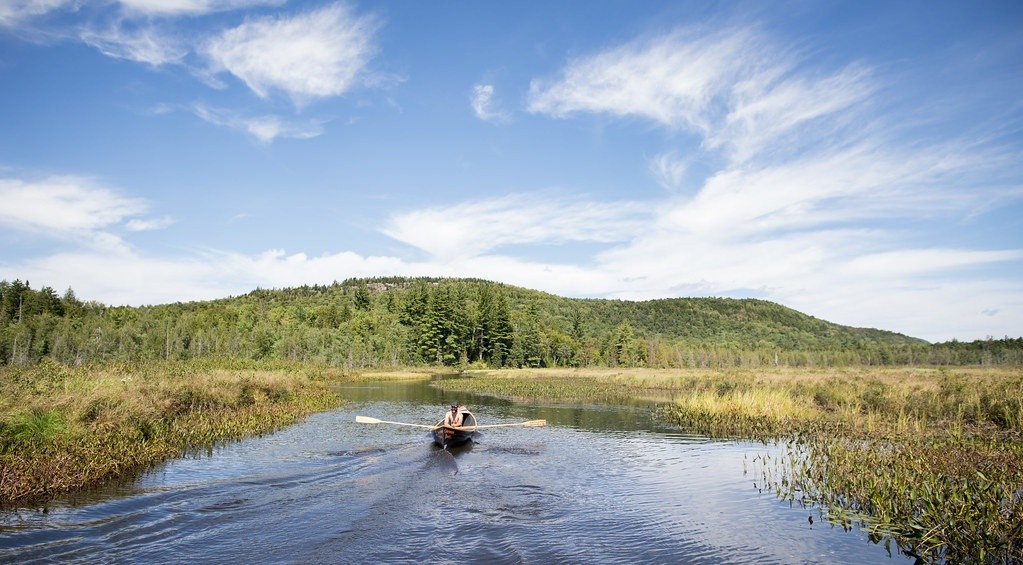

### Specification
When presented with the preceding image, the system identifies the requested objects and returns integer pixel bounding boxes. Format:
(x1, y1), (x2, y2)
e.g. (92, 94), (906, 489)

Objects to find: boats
(430, 404), (479, 449)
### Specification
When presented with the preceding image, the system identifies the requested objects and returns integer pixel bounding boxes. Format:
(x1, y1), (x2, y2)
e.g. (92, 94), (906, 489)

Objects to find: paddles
(354, 415), (436, 428)
(455, 420), (547, 429)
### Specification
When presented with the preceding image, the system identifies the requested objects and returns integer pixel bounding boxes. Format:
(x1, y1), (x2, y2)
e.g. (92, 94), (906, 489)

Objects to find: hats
(451, 402), (459, 408)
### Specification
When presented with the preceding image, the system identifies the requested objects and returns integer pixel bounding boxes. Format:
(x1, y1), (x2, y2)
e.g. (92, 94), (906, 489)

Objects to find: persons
(444, 401), (464, 427)
